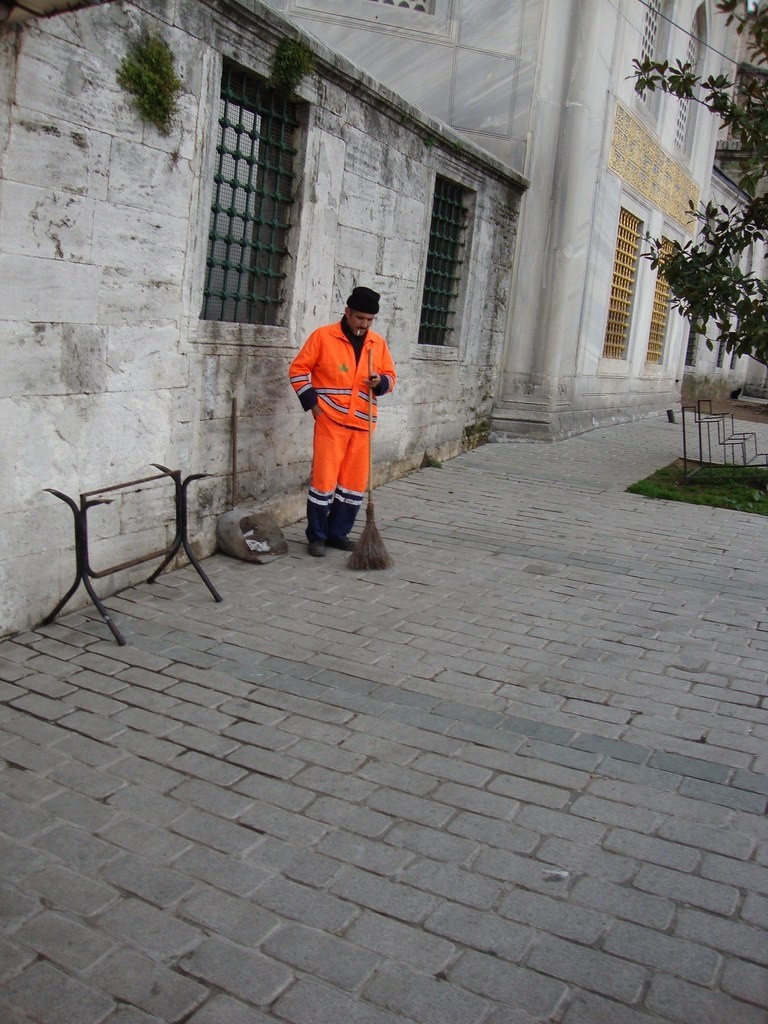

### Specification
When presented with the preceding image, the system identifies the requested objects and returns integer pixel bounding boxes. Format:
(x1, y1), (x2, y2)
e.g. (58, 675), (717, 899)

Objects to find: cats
(731, 388), (742, 399)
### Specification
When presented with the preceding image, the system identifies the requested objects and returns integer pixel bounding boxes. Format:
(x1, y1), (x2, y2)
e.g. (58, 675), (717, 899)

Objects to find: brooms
(345, 348), (395, 572)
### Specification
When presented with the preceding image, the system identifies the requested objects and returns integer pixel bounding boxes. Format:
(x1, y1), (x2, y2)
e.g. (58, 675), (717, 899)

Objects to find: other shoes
(326, 535), (358, 551)
(307, 539), (327, 556)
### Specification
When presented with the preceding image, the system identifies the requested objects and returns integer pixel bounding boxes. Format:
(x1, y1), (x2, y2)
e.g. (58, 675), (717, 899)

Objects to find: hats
(347, 286), (380, 314)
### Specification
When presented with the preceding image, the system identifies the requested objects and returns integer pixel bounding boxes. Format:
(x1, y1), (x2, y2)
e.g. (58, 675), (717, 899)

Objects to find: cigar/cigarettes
(356, 328), (360, 335)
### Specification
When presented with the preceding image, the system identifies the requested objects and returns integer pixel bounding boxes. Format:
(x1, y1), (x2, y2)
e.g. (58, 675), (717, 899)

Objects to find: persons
(289, 285), (397, 556)
